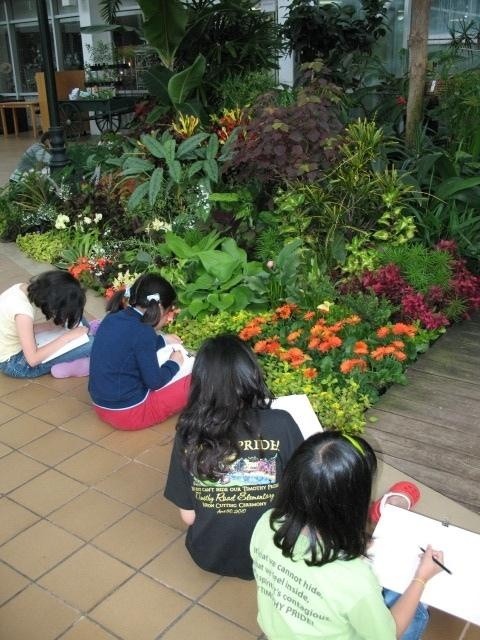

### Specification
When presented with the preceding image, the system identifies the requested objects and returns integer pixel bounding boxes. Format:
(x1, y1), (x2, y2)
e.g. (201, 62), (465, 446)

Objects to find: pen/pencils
(418, 545), (453, 576)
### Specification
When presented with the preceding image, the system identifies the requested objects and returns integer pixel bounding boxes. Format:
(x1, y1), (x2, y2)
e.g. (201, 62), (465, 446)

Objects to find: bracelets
(60, 337), (68, 343)
(412, 578), (425, 585)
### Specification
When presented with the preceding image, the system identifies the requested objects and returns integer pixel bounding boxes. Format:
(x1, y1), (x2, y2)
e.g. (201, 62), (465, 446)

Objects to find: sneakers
(50, 357), (91, 379)
(89, 319), (101, 336)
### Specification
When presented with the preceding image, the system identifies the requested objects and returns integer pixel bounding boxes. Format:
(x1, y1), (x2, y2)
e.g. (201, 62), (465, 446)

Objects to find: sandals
(369, 480), (420, 524)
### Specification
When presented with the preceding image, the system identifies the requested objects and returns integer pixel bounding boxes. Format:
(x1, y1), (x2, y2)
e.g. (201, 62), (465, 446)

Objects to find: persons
(88, 273), (193, 431)
(9, 132), (52, 183)
(0, 270), (101, 379)
(249, 430), (444, 640)
(162, 334), (304, 581)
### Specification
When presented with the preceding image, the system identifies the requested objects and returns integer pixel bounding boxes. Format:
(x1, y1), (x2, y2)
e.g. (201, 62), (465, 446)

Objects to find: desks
(0, 101), (42, 139)
